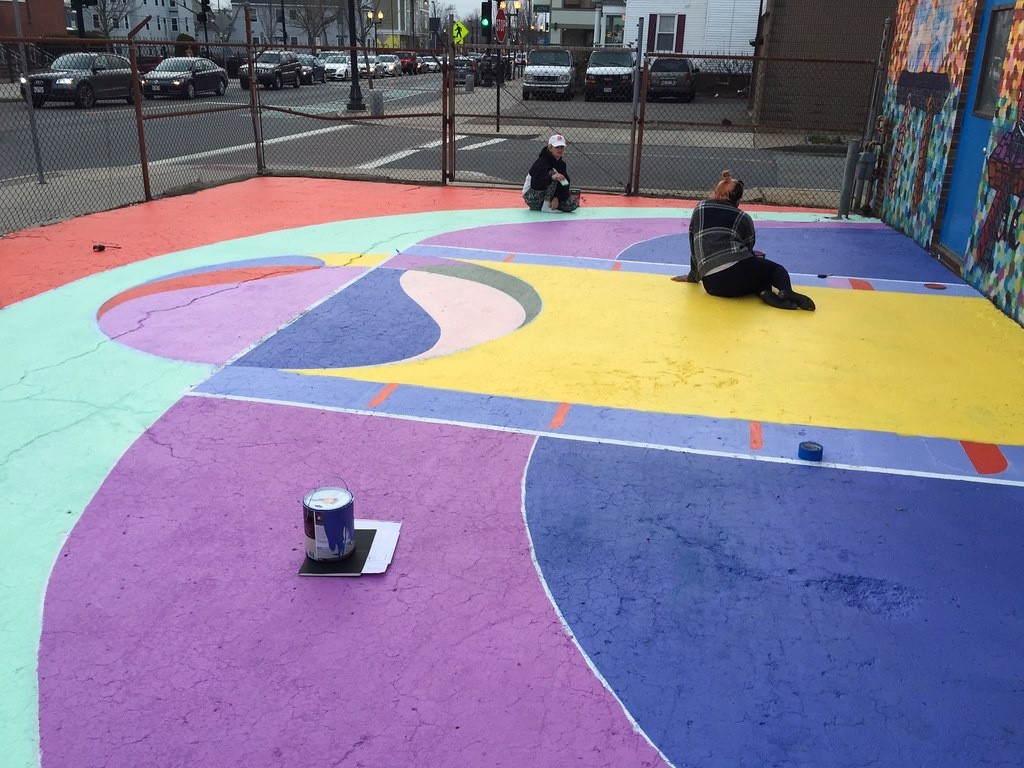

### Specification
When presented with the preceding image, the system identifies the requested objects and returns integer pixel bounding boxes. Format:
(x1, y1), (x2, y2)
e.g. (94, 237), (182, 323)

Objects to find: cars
(139, 57), (228, 100)
(358, 56), (386, 78)
(20, 53), (143, 109)
(326, 58), (352, 80)
(296, 52), (327, 84)
(438, 57), (449, 69)
(378, 55), (402, 77)
(317, 51), (345, 65)
(416, 57), (427, 75)
(425, 57), (441, 72)
(454, 51), (526, 86)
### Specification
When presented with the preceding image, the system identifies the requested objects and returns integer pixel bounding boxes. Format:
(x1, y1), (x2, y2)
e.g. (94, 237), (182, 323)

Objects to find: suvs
(445, 58), (478, 88)
(238, 49), (303, 91)
(397, 52), (418, 75)
(648, 57), (701, 102)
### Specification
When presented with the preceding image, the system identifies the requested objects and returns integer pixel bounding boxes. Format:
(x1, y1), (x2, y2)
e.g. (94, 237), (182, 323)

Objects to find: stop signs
(495, 8), (506, 43)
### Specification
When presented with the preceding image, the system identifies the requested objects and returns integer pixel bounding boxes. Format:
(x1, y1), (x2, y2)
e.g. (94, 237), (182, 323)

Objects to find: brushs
(553, 167), (569, 186)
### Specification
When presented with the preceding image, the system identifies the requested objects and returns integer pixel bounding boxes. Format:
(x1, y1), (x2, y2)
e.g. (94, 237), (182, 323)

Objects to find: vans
(522, 49), (579, 101)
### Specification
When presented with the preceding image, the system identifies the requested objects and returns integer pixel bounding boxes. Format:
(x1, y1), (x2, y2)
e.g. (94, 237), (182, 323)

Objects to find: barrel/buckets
(302, 476), (356, 560)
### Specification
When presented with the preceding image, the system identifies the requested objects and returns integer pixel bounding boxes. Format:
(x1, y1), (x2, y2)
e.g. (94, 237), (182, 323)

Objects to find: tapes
(798, 440), (824, 461)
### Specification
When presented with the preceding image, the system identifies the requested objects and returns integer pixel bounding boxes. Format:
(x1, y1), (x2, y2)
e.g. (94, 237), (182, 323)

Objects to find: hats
(548, 135), (566, 148)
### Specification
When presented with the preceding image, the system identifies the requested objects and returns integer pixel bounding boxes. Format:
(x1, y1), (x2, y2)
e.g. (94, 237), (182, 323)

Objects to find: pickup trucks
(584, 50), (637, 103)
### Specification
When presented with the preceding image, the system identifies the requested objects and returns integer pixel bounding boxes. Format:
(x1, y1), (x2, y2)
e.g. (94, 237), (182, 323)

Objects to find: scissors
(818, 273), (833, 278)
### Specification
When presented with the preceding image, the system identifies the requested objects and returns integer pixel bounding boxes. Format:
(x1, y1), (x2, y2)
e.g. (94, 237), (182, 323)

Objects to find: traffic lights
(85, 0), (98, 8)
(201, 0), (211, 13)
(481, 2), (491, 37)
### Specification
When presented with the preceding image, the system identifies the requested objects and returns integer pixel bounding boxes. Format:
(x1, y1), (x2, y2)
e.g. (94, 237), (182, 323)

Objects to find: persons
(670, 170), (815, 311)
(521, 135), (570, 213)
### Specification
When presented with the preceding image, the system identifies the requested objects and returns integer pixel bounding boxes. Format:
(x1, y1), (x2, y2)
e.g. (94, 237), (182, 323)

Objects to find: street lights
(368, 10), (384, 57)
(499, 0), (521, 81)
